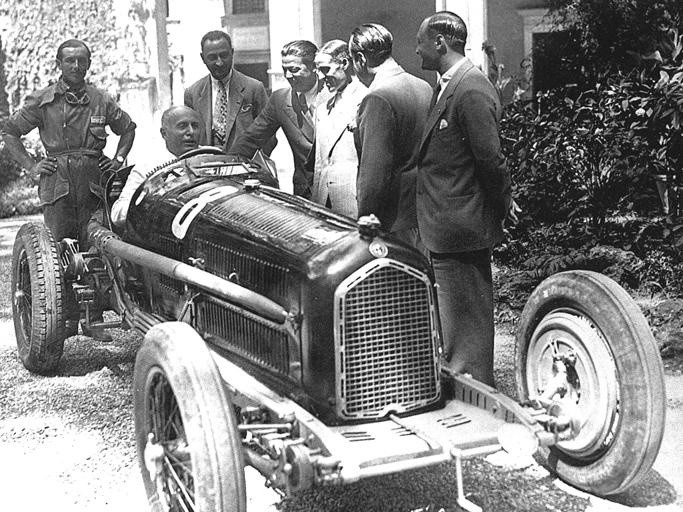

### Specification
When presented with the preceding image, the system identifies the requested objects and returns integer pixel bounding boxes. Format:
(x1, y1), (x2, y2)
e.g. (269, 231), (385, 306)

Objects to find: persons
(348, 24), (433, 261)
(3, 39), (137, 252)
(415, 12), (522, 389)
(110, 106), (202, 230)
(227, 24), (368, 221)
(183, 31), (278, 159)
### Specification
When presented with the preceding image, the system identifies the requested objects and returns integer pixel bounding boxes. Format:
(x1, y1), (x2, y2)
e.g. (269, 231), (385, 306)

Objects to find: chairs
(104, 164), (136, 236)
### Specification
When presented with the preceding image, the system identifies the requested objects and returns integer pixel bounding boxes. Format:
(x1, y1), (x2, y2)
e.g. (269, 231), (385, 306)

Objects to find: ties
(299, 94), (308, 114)
(213, 81), (228, 144)
(428, 78), (441, 116)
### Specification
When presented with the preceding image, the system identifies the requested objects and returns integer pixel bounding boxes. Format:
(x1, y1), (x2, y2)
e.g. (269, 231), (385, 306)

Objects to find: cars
(11, 145), (667, 512)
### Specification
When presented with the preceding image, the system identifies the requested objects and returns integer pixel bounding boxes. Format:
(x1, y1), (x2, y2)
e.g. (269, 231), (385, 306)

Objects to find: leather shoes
(64, 318), (112, 342)
